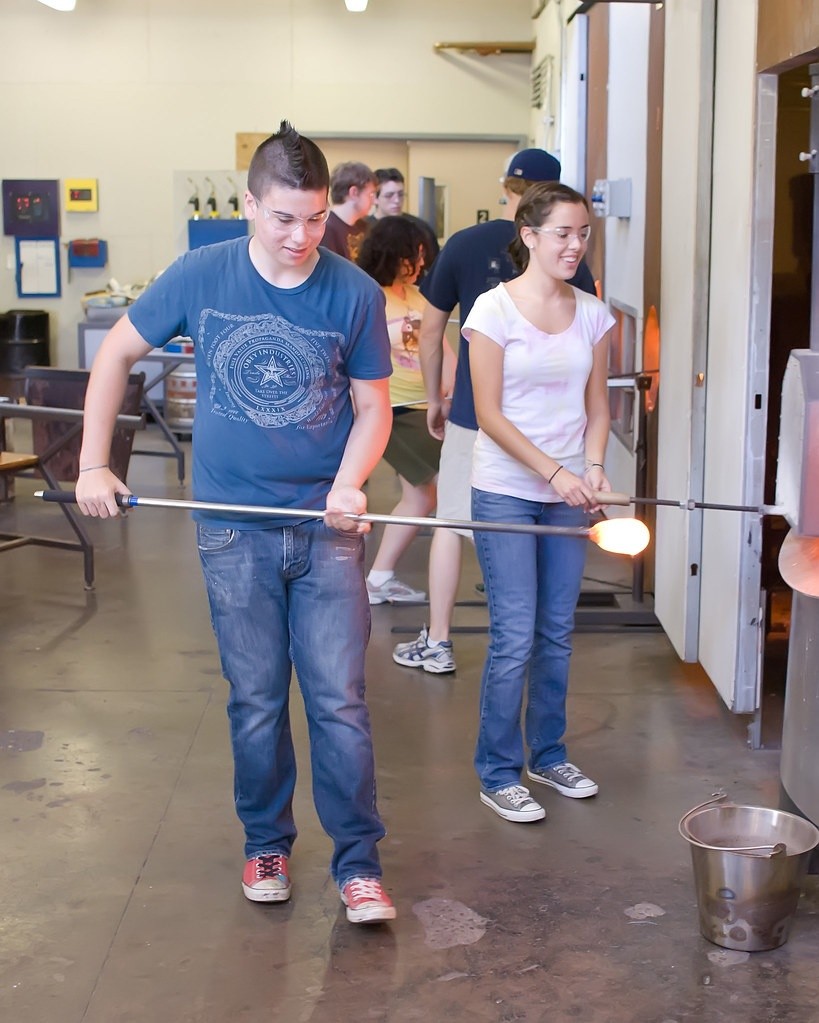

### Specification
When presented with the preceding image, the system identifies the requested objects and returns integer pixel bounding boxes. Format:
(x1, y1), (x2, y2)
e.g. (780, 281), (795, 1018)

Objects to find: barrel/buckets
(678, 787), (819, 951)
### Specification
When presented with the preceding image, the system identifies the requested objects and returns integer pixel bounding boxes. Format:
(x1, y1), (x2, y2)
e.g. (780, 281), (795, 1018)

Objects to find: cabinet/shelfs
(77, 319), (165, 417)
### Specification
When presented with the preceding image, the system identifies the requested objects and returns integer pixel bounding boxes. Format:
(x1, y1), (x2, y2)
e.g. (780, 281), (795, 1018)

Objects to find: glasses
(530, 226), (592, 244)
(254, 198), (331, 231)
(357, 190), (376, 200)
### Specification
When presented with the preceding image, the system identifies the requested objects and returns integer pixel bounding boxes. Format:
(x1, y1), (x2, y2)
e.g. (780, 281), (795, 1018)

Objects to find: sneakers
(527, 763), (599, 799)
(393, 623), (457, 674)
(242, 854), (291, 903)
(366, 576), (427, 605)
(339, 876), (396, 925)
(478, 785), (546, 822)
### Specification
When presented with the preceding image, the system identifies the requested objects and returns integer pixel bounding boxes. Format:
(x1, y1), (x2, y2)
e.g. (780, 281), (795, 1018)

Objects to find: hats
(506, 148), (562, 182)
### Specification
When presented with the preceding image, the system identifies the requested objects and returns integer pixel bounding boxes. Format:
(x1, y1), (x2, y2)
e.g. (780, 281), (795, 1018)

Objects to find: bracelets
(79, 464), (107, 472)
(593, 464), (604, 469)
(548, 466), (563, 483)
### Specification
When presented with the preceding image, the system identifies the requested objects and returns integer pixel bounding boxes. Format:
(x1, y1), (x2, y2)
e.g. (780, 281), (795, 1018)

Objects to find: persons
(76, 120), (397, 924)
(353, 215), (458, 605)
(460, 182), (616, 822)
(320, 160), (406, 261)
(391, 148), (598, 674)
